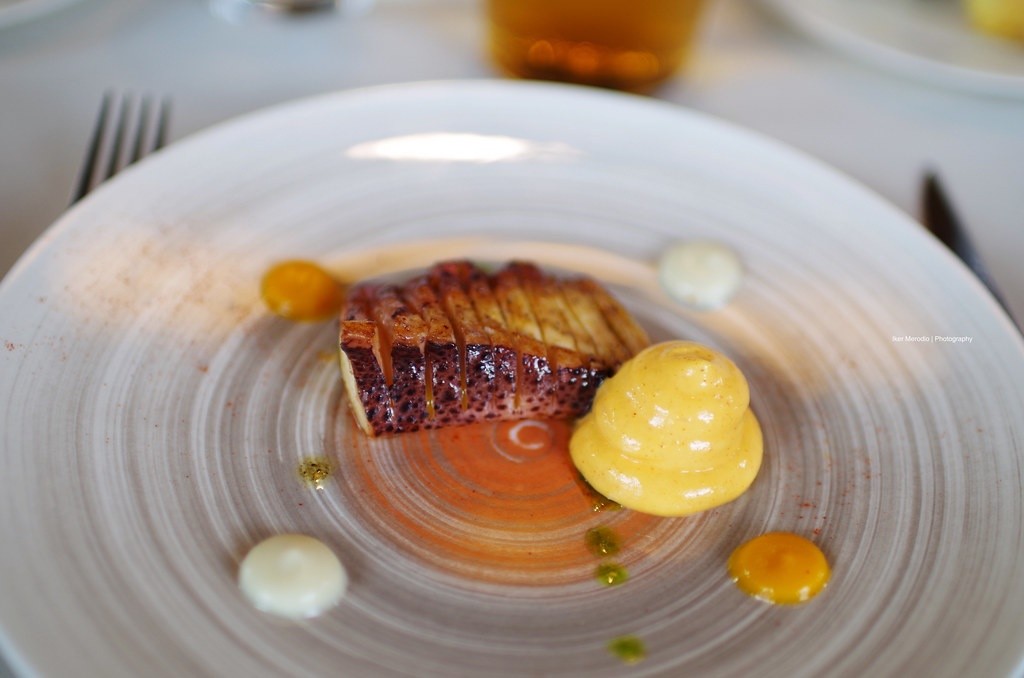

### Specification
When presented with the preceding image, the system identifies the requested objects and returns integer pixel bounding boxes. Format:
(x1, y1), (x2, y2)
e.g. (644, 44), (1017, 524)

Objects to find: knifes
(920, 168), (1023, 341)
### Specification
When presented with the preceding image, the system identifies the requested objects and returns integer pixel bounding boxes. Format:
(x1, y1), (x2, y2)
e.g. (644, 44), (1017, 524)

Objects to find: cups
(473, 0), (711, 97)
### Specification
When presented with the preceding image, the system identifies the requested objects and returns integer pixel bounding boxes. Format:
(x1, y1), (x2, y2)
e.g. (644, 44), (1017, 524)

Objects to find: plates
(760, 0), (1024, 103)
(0, 81), (1023, 678)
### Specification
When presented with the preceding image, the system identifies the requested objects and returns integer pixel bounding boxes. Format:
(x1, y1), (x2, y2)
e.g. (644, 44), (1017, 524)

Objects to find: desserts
(568, 338), (765, 520)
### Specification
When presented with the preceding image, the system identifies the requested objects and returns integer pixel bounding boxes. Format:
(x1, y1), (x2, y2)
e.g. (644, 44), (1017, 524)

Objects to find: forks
(57, 89), (176, 212)
